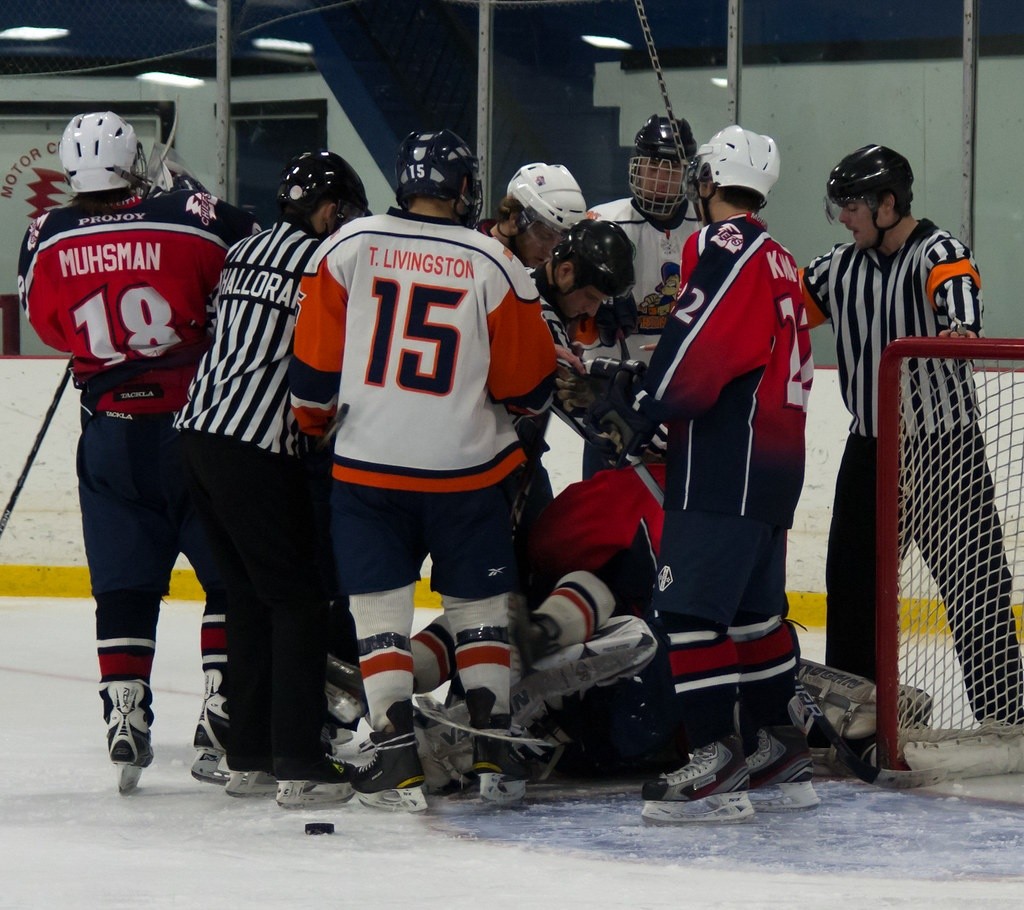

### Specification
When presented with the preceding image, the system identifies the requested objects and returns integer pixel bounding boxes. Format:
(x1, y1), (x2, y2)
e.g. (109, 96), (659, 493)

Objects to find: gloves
(554, 360), (591, 416)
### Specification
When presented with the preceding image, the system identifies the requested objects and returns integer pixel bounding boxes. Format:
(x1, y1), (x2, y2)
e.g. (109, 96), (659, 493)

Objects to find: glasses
(526, 219), (565, 246)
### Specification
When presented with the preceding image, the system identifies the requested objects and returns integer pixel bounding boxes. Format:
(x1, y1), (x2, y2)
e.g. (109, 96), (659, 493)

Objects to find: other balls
(305, 823), (334, 835)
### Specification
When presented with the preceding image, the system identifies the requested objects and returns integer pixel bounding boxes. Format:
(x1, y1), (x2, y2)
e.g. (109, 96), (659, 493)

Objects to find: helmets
(633, 114), (697, 163)
(274, 147), (370, 226)
(693, 124), (782, 201)
(558, 218), (638, 301)
(57, 110), (140, 193)
(826, 144), (913, 211)
(393, 127), (481, 200)
(506, 161), (587, 232)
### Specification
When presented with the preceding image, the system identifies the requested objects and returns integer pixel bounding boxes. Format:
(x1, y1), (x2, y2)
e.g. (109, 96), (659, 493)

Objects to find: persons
(15, 109), (260, 794)
(476, 159), (588, 267)
(402, 460), (690, 695)
(165, 148), (381, 809)
(509, 217), (637, 566)
(629, 126), (822, 821)
(793, 143), (1024, 729)
(289, 127), (563, 814)
(585, 115), (720, 366)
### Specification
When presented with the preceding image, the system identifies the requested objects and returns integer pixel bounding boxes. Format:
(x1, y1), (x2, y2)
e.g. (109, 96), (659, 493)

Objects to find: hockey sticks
(0, 92), (180, 537)
(617, 328), (631, 364)
(632, 0), (702, 221)
(552, 402), (623, 453)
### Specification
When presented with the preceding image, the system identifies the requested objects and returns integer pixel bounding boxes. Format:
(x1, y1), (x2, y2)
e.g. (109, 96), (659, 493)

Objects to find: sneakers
(189, 668), (229, 786)
(348, 697), (428, 814)
(219, 750), (279, 800)
(464, 686), (534, 804)
(273, 751), (358, 811)
(741, 723), (819, 813)
(641, 731), (754, 826)
(99, 678), (154, 796)
